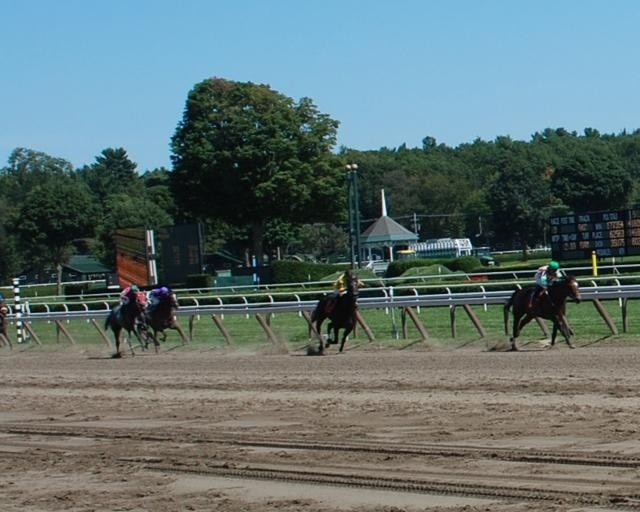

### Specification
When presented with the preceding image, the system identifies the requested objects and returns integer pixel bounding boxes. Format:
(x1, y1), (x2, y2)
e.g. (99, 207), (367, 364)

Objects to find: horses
(105, 290), (189, 359)
(0, 304), (13, 352)
(310, 269), (360, 353)
(503, 276), (582, 351)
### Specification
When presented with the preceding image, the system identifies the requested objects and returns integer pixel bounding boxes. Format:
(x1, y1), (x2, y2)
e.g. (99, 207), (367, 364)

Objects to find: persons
(0, 292), (10, 315)
(534, 260), (559, 302)
(147, 285), (170, 309)
(332, 270), (364, 308)
(120, 284), (150, 347)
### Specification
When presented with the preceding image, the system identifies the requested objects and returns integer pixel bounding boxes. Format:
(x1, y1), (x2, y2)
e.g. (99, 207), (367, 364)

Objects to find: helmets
(161, 286), (169, 294)
(131, 284), (138, 292)
(549, 260), (560, 269)
